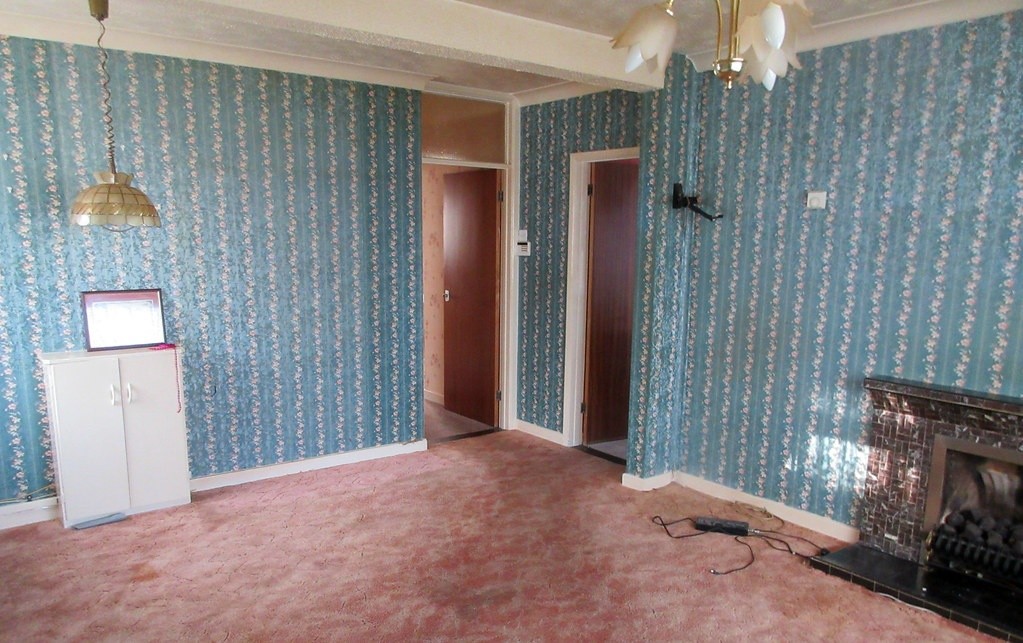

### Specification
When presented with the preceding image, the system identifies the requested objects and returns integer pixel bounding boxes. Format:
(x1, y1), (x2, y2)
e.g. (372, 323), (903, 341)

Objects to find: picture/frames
(82, 288), (168, 352)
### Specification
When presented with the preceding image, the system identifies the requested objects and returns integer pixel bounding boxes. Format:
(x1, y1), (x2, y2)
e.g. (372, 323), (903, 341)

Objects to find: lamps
(607, 0), (814, 91)
(68, 0), (161, 232)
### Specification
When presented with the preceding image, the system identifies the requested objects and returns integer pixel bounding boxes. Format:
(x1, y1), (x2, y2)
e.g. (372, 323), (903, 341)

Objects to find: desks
(37, 343), (192, 529)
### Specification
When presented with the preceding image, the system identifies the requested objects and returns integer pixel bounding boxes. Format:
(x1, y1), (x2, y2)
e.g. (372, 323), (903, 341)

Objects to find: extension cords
(694, 516), (749, 536)
